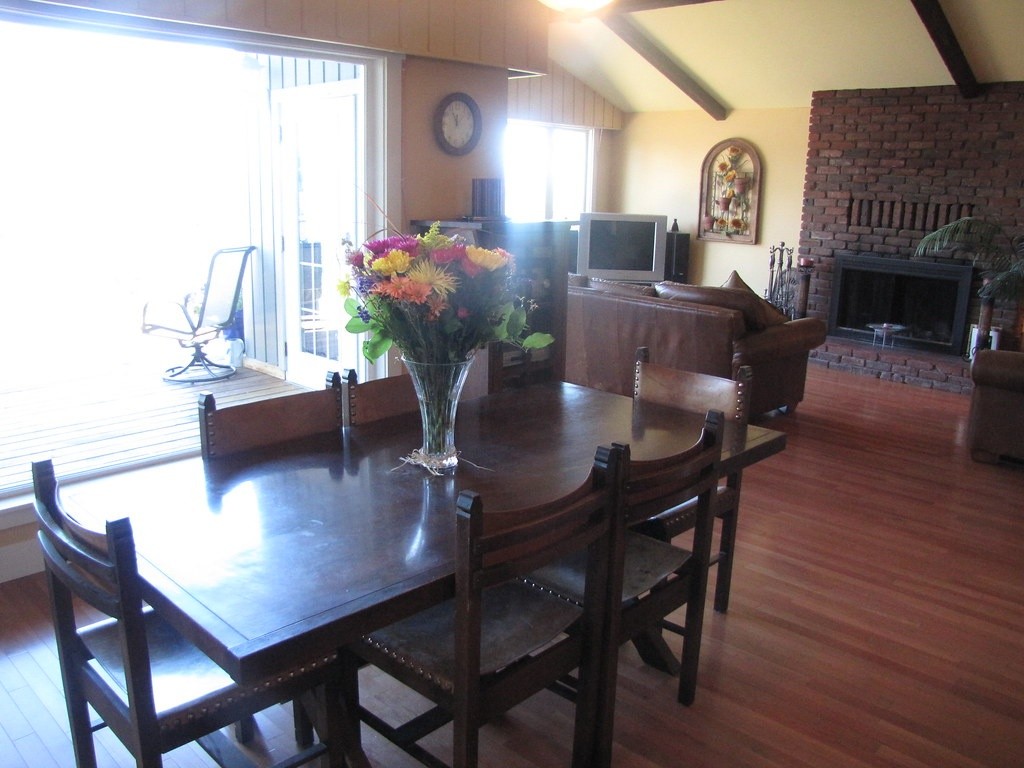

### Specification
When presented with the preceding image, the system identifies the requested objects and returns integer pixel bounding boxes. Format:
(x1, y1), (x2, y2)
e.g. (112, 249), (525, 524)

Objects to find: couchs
(963, 350), (1024, 464)
(563, 265), (828, 421)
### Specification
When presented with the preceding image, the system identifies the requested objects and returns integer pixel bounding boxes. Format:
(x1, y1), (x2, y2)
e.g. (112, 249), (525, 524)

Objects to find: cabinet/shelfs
(410, 219), (579, 396)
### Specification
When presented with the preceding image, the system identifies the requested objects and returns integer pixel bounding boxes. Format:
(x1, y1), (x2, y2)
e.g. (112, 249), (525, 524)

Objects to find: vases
(399, 351), (475, 473)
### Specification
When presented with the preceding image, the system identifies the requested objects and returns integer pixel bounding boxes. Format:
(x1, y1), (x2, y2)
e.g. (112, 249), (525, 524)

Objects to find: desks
(59, 377), (792, 768)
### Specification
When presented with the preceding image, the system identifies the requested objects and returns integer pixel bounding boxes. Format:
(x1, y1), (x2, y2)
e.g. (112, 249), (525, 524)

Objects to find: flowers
(342, 223), (548, 371)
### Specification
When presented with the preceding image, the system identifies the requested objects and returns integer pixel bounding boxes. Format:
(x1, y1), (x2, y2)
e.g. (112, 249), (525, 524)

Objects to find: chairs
(138, 243), (258, 385)
(342, 367), (430, 427)
(520, 406), (735, 768)
(345, 432), (630, 767)
(198, 370), (342, 453)
(626, 347), (757, 611)
(32, 450), (361, 768)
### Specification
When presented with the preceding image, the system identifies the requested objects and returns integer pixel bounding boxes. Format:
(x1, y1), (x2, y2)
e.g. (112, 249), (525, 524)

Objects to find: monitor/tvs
(577, 212), (668, 285)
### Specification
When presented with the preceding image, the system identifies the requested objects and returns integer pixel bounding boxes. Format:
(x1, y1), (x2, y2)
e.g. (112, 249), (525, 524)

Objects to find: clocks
(431, 91), (484, 154)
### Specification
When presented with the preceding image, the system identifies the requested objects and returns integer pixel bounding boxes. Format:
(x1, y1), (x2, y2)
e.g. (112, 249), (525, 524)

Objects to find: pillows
(721, 270), (790, 332)
